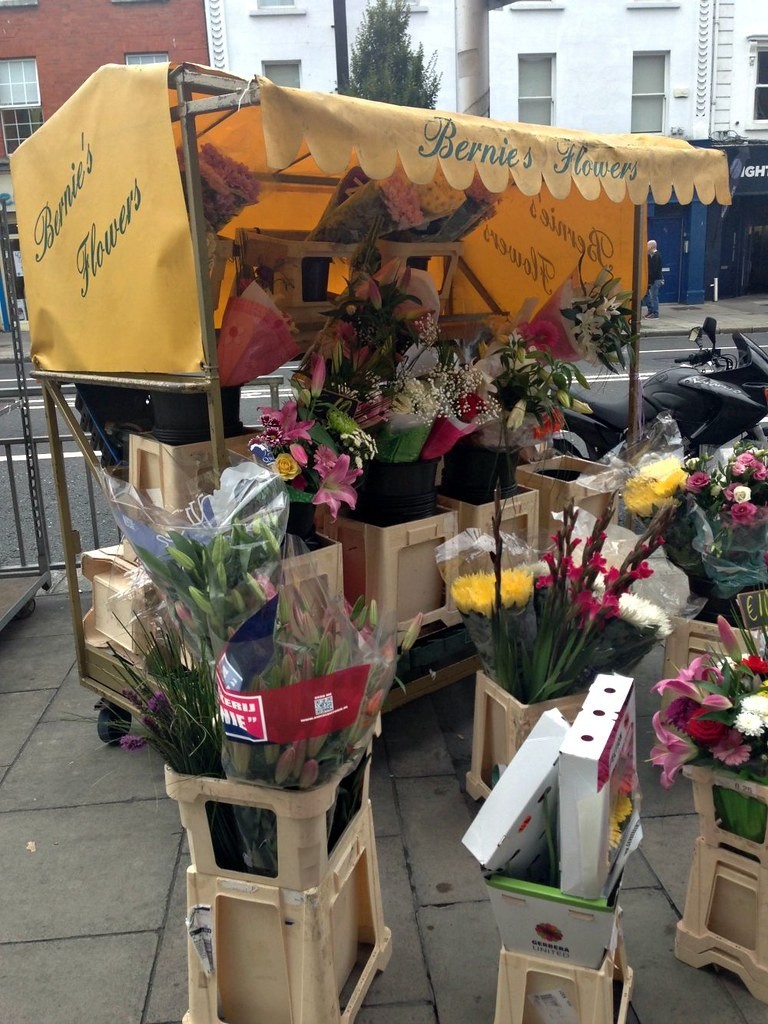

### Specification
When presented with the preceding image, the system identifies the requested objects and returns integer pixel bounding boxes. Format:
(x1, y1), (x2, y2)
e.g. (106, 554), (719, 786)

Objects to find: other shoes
(644, 312), (660, 320)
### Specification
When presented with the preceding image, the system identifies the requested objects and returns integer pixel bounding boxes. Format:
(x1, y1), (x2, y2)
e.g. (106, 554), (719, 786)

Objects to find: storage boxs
(279, 531), (346, 645)
(558, 672), (640, 899)
(437, 482), (541, 578)
(317, 505), (463, 643)
(121, 427), (261, 568)
(514, 454), (620, 557)
(83, 543), (160, 672)
(218, 864), (360, 1024)
(460, 706), (570, 880)
(90, 569), (151, 654)
(523, 971), (578, 1024)
(481, 866), (626, 968)
(708, 862), (758, 958)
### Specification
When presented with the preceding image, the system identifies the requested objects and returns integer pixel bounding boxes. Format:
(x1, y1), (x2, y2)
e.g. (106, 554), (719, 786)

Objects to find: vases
(342, 456), (443, 527)
(287, 501), (317, 539)
(440, 434), (520, 503)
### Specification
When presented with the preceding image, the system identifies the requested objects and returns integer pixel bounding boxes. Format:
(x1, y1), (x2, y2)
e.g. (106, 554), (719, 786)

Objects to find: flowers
(57, 142), (768, 886)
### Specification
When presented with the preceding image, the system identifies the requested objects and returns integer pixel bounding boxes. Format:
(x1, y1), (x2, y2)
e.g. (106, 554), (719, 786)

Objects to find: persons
(644, 239), (662, 320)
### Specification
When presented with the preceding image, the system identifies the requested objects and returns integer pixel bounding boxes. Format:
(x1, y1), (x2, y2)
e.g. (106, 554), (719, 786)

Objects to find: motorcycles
(553, 316), (767, 462)
